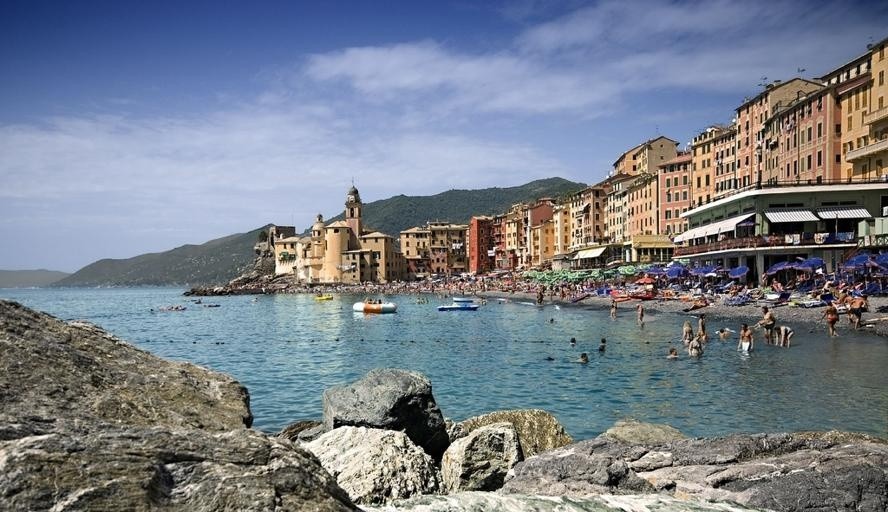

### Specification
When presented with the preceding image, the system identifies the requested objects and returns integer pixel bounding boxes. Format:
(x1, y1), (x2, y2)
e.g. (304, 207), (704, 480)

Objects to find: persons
(364, 296), (382, 304)
(569, 284), (869, 362)
(537, 285), (583, 305)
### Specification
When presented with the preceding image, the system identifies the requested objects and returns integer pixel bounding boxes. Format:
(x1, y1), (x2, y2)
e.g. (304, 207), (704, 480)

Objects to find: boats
(352, 301), (399, 312)
(433, 293), (489, 311)
(354, 311), (396, 322)
(314, 290), (333, 300)
